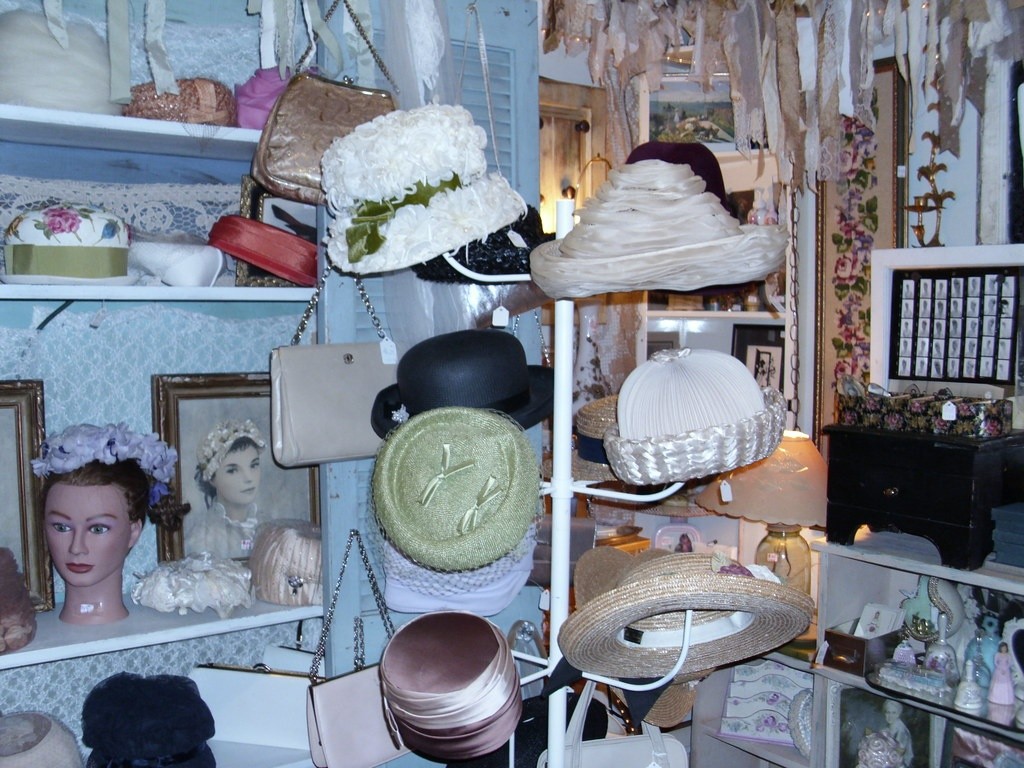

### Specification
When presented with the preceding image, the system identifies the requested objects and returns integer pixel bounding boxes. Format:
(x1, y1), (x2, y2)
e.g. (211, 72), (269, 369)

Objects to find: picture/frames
(647, 71), (743, 153)
(730, 323), (787, 399)
(150, 370), (324, 569)
(0, 377), (58, 616)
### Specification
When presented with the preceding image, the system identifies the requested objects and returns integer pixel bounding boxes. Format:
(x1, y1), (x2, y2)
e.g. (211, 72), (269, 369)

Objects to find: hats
(322, 103), (528, 274)
(528, 139), (790, 298)
(0, 712), (85, 768)
(571, 347), (787, 516)
(557, 547), (816, 728)
(378, 609), (522, 760)
(234, 65), (318, 129)
(195, 419), (265, 482)
(81, 671), (216, 768)
(370, 328), (555, 617)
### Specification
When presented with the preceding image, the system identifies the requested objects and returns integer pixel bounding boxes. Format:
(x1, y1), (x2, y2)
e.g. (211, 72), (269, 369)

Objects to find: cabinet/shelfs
(808, 526), (1024, 768)
(0, 0), (337, 767)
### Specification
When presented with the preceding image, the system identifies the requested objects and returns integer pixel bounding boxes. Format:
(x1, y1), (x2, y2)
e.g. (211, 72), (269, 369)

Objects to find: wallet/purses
(187, 663), (326, 752)
(207, 215), (317, 288)
(122, 78), (239, 129)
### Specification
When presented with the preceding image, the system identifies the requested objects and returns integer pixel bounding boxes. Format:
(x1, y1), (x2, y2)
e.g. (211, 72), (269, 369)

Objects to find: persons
(30, 421), (178, 625)
(180, 418), (269, 559)
(986, 641), (1014, 705)
(877, 699), (914, 768)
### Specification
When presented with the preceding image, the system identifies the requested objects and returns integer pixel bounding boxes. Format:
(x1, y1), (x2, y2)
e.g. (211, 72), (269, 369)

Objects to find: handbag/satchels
(270, 261), (399, 468)
(305, 529), (411, 768)
(249, 0), (397, 206)
(446, 621), (686, 768)
(412, 6), (556, 329)
(524, 495), (597, 587)
(268, 616), (325, 677)
